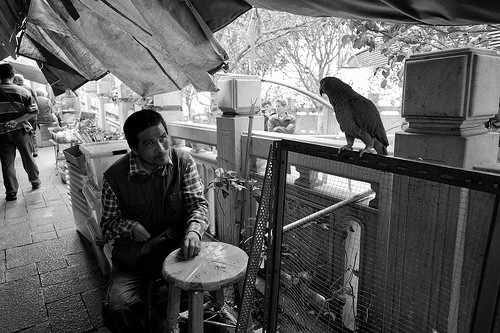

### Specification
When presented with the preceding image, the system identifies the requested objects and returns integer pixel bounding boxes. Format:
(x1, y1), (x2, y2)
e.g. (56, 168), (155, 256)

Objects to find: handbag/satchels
(20, 120), (33, 133)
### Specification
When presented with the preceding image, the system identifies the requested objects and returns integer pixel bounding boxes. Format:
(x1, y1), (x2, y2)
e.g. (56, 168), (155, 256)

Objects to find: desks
(48, 138), (71, 170)
(161, 242), (254, 333)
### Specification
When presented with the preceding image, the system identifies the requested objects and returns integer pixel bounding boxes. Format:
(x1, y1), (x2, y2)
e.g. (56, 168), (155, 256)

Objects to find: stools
(37, 121), (58, 148)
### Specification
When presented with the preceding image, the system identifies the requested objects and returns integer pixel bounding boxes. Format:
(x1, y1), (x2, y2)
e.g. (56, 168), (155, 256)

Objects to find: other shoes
(33, 150), (38, 157)
(32, 180), (41, 189)
(5, 192), (17, 201)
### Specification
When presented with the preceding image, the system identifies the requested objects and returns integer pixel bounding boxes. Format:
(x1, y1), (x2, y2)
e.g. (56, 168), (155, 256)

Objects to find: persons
(99, 109), (228, 333)
(0, 63), (42, 201)
(11, 73), (40, 156)
(267, 98), (296, 134)
(261, 101), (276, 132)
(33, 88), (61, 128)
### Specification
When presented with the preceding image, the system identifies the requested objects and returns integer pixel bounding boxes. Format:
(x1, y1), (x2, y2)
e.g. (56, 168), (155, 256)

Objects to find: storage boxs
(63, 140), (132, 277)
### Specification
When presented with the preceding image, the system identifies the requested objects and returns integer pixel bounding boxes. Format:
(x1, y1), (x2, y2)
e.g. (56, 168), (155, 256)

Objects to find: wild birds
(318, 76), (389, 158)
